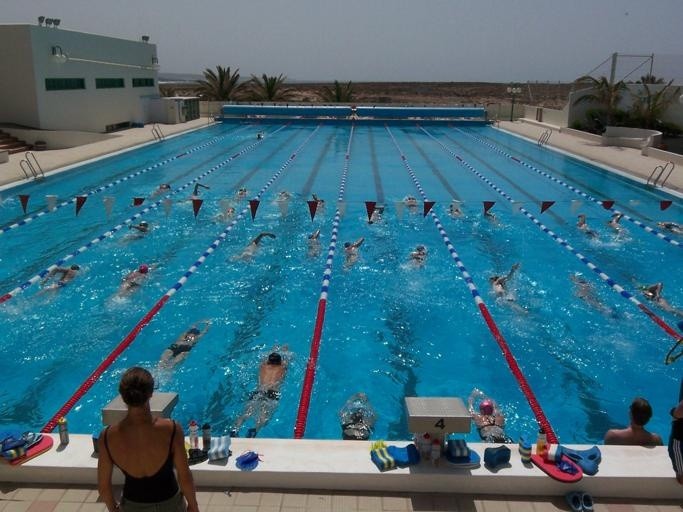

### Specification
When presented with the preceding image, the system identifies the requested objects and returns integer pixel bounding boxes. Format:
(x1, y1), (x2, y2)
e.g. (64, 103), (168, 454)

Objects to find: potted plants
(34, 141), (46, 151)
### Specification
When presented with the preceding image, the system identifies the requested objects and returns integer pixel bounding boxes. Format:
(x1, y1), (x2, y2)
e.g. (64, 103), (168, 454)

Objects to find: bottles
(57, 417), (69, 446)
(432, 440), (440, 469)
(189, 420), (199, 449)
(201, 422), (210, 449)
(537, 429), (545, 455)
(518, 433), (531, 464)
(421, 432), (430, 460)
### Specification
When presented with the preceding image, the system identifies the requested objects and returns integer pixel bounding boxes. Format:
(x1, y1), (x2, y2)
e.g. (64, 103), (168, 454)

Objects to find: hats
(268, 352), (281, 365)
(140, 220), (148, 227)
(479, 399), (494, 415)
(71, 264), (79, 271)
(226, 208), (234, 213)
(139, 264), (149, 273)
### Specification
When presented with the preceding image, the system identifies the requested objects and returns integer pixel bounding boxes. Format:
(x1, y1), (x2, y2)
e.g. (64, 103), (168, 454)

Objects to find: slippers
(565, 490), (582, 512)
(577, 490), (594, 512)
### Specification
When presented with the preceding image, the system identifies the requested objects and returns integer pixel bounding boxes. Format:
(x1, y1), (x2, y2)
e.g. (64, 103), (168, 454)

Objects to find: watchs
(669, 407), (680, 419)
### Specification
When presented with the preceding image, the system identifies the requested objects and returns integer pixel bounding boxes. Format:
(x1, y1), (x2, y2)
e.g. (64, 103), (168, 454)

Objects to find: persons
(668, 378), (683, 485)
(469, 387), (505, 443)
(97, 366), (199, 512)
(605, 397), (663, 446)
(643, 282), (683, 316)
(156, 321), (208, 385)
(489, 263), (527, 315)
(28, 264), (79, 299)
(257, 131), (265, 141)
(657, 221), (683, 235)
(230, 344), (289, 438)
(576, 213), (599, 239)
(115, 264), (158, 297)
(339, 392), (376, 441)
(608, 213), (629, 242)
(571, 276), (609, 314)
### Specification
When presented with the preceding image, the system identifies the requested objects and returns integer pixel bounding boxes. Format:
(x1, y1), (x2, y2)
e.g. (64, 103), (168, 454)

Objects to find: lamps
(49, 45), (68, 64)
